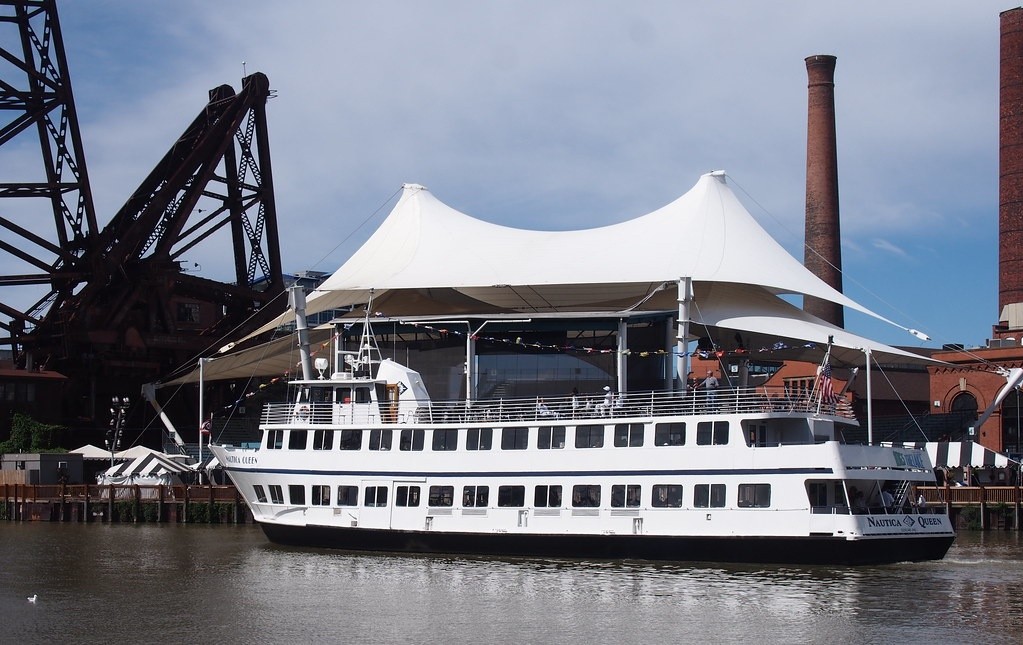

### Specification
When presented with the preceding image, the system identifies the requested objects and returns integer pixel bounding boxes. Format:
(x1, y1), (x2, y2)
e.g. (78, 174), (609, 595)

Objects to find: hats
(706, 370), (713, 374)
(602, 385), (610, 391)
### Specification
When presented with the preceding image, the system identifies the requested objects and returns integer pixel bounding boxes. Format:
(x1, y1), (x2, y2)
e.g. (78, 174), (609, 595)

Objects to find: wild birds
(27, 594), (38, 605)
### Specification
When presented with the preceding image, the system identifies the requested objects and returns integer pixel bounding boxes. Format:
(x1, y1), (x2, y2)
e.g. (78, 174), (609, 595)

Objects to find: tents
(96, 452), (194, 498)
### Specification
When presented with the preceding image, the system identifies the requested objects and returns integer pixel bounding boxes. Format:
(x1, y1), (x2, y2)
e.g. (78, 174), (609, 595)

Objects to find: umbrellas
(111, 445), (167, 461)
(68, 444), (112, 461)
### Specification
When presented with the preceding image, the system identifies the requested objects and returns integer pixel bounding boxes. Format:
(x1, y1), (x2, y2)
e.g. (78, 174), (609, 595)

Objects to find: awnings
(874, 441), (1020, 469)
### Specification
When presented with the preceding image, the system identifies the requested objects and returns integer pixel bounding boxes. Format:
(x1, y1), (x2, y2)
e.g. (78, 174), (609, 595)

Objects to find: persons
(848, 486), (864, 513)
(245, 443), (249, 448)
(579, 398), (592, 413)
(539, 397), (550, 417)
(595, 386), (612, 419)
(687, 370), (719, 415)
(571, 389), (580, 419)
(917, 493), (925, 514)
(882, 487), (895, 514)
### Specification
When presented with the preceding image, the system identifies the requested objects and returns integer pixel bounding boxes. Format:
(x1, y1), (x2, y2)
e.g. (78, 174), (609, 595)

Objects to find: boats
(140, 169), (1023, 566)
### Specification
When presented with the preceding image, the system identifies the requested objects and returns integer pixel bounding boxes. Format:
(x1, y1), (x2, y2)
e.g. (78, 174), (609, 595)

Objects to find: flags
(820, 356), (839, 405)
(200, 419), (212, 432)
